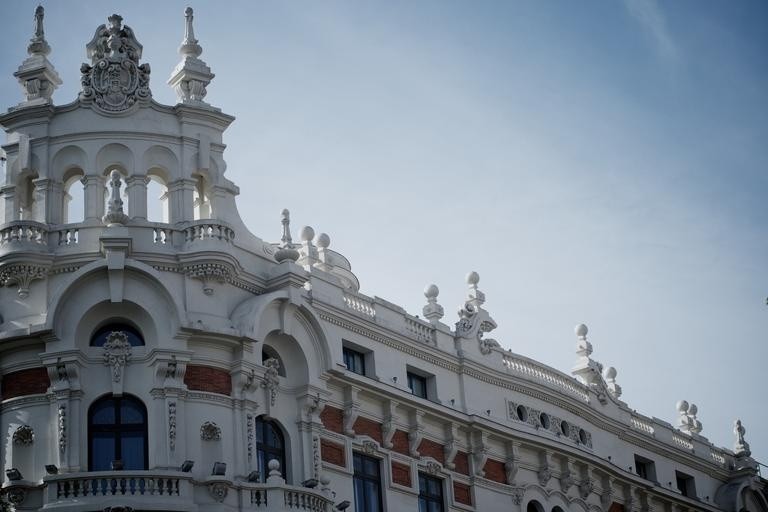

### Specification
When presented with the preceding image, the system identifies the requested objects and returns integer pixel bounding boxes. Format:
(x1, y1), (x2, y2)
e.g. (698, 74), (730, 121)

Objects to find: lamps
(182, 460), (195, 472)
(213, 462), (226, 476)
(246, 471), (261, 482)
(302, 479), (319, 488)
(336, 501), (351, 511)
(45, 464), (58, 475)
(5, 468), (24, 481)
(111, 459), (124, 470)
